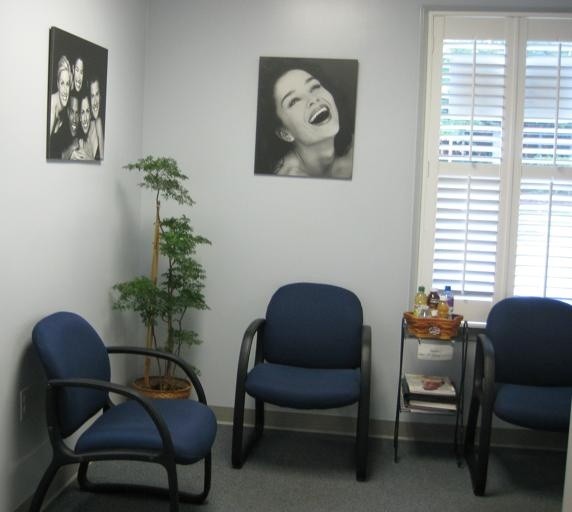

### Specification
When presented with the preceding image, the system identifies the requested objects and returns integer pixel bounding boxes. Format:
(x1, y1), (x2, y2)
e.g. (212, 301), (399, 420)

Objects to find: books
(402, 373), (459, 416)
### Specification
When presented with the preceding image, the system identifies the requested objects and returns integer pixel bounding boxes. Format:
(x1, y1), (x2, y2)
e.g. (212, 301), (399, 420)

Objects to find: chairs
(30, 307), (217, 511)
(462, 292), (569, 497)
(230, 282), (373, 483)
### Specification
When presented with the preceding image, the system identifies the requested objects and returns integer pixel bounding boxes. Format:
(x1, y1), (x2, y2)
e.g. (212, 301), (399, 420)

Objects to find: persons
(256, 59), (355, 180)
(49, 49), (105, 163)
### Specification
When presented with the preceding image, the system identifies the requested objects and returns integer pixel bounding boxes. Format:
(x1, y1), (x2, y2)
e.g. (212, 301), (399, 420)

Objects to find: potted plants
(113, 152), (207, 403)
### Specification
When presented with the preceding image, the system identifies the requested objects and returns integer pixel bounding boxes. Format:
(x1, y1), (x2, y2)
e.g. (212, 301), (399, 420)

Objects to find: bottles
(414, 284), (455, 319)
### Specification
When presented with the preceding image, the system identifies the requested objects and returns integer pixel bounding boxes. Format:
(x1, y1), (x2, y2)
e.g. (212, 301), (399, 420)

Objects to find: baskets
(404, 311), (463, 339)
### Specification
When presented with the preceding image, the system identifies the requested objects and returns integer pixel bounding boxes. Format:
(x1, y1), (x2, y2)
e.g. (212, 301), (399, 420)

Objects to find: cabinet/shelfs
(394, 316), (470, 467)
